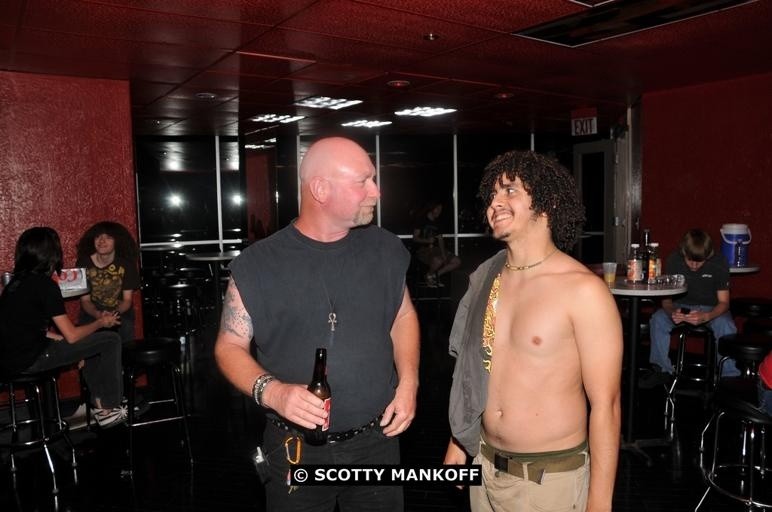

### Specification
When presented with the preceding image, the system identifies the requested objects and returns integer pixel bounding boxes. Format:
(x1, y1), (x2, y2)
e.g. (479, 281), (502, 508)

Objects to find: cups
(602, 262), (617, 286)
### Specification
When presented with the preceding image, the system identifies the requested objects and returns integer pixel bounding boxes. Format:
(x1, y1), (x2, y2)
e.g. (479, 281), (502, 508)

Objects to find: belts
(272, 416), (381, 446)
(480, 444), (585, 484)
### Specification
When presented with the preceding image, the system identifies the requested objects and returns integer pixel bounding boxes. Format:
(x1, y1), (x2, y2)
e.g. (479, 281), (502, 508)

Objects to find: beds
(601, 276), (688, 468)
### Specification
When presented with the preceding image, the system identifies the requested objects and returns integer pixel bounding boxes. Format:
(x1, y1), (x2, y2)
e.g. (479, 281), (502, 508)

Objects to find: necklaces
(321, 280), (341, 332)
(504, 247), (559, 270)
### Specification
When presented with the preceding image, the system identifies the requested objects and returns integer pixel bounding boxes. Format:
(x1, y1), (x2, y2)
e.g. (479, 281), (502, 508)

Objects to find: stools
(119, 338), (195, 478)
(643, 300), (772, 512)
(1, 336), (77, 496)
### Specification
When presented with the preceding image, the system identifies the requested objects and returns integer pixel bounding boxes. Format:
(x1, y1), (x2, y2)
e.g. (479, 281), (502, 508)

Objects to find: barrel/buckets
(719, 224), (753, 267)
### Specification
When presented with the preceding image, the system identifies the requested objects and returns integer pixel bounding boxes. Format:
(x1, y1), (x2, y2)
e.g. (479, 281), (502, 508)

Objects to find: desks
(730, 266), (759, 275)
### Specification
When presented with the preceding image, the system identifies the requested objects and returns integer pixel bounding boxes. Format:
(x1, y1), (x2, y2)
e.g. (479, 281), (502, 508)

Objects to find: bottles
(735, 239), (744, 267)
(304, 349), (332, 446)
(626, 228), (686, 288)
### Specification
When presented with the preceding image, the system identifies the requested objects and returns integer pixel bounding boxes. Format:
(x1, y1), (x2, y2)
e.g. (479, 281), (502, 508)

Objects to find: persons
(1, 224), (150, 430)
(76, 220), (142, 344)
(413, 200), (462, 289)
(638, 227), (742, 390)
(442, 149), (625, 512)
(213, 135), (423, 511)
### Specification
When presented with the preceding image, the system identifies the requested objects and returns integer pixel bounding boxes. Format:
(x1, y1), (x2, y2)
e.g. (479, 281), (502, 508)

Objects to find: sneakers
(640, 370), (673, 388)
(90, 397), (149, 427)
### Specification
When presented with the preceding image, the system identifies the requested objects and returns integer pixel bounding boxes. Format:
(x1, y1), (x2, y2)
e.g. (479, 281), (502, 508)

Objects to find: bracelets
(250, 372), (276, 409)
